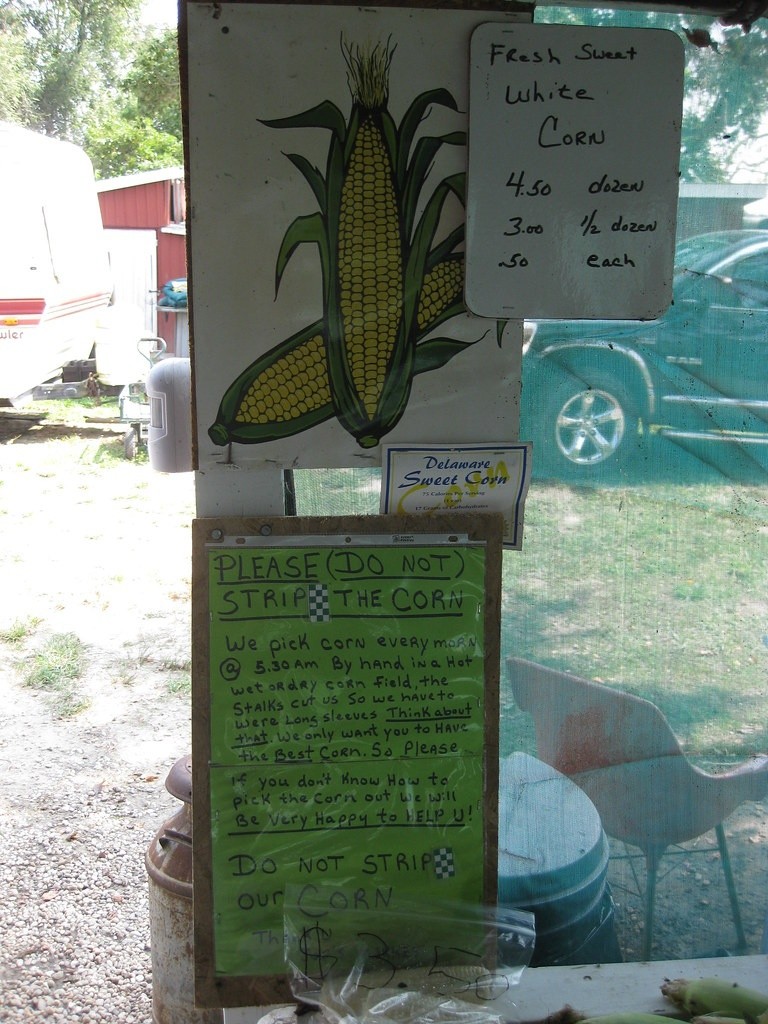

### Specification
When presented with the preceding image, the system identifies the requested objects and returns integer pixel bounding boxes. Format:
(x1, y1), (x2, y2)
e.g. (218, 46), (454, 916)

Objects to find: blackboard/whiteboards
(462, 20), (684, 320)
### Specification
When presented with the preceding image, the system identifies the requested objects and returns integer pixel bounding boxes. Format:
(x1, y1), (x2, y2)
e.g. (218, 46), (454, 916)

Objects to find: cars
(521, 227), (766, 482)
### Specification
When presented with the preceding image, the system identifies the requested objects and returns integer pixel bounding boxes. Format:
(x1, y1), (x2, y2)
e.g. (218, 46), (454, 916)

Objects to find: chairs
(505, 656), (768, 961)
